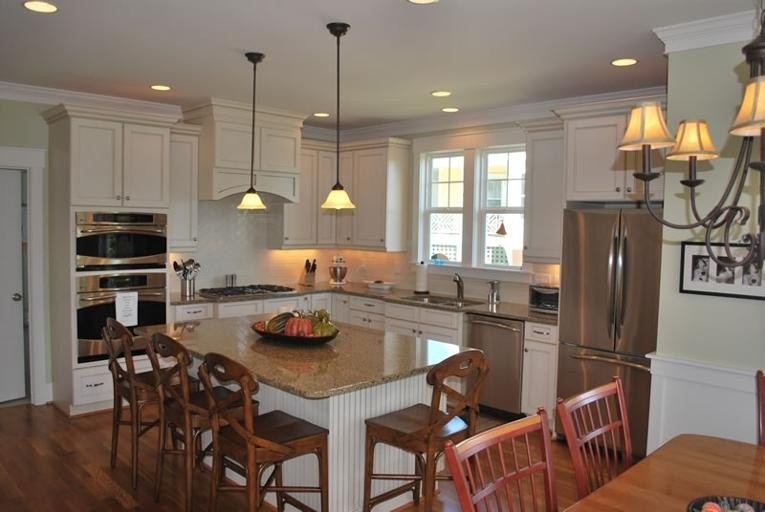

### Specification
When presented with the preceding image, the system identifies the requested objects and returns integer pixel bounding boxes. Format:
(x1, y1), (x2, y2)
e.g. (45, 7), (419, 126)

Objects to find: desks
(160, 311), (485, 512)
(557, 433), (765, 512)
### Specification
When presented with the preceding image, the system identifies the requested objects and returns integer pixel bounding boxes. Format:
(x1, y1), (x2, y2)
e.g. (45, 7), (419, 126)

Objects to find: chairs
(195, 348), (331, 511)
(554, 372), (643, 496)
(441, 405), (554, 512)
(101, 321), (201, 491)
(756, 365), (765, 448)
(359, 346), (492, 512)
(146, 333), (260, 511)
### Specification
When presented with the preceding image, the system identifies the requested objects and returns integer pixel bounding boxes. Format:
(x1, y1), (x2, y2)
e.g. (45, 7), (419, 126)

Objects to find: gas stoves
(200, 283), (292, 301)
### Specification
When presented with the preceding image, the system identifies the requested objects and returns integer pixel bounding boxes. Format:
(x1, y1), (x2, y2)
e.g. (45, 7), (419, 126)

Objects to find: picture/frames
(677, 237), (764, 299)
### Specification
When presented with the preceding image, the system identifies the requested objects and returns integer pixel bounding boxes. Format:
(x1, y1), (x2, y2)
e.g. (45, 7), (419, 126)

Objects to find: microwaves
(528, 284), (559, 314)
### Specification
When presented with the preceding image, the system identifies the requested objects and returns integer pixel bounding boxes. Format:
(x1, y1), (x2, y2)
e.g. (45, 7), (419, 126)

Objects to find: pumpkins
(267, 309), (335, 336)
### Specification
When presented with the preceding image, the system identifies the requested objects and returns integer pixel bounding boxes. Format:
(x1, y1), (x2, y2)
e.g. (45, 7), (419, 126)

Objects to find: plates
(250, 337), (340, 362)
(687, 495), (764, 511)
(249, 317), (341, 345)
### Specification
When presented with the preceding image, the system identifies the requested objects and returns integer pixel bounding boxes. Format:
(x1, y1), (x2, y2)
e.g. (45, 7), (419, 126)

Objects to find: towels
(114, 291), (139, 327)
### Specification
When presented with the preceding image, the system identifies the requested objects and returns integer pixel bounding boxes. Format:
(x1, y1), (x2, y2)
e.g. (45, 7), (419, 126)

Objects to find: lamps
(234, 49), (275, 213)
(324, 21), (363, 213)
(617, 2), (764, 267)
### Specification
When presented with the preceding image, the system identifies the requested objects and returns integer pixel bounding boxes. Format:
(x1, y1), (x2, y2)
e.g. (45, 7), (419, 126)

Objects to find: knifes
(305, 258), (317, 273)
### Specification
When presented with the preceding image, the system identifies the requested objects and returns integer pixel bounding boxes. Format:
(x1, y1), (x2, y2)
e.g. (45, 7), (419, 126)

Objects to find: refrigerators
(555, 200), (664, 457)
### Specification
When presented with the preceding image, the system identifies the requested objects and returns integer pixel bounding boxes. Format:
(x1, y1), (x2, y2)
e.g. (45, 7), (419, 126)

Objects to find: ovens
(75, 211), (168, 364)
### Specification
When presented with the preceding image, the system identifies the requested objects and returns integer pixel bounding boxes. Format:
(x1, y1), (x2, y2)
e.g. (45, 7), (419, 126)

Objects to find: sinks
(398, 294), (485, 309)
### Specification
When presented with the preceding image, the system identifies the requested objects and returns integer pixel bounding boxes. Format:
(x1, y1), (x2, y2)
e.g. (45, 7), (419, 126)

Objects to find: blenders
(328, 255), (348, 285)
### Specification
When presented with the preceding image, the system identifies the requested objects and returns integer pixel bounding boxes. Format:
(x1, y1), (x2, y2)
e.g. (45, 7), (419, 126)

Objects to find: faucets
(453, 273), (464, 299)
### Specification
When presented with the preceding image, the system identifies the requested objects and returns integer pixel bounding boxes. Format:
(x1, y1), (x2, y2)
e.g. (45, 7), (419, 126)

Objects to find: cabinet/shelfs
(521, 318), (558, 431)
(266, 134), (338, 249)
(305, 293), (332, 320)
(339, 136), (414, 252)
(170, 304), (212, 324)
(551, 87), (666, 203)
(213, 296), (304, 318)
(168, 125), (199, 253)
(385, 297), (466, 347)
(333, 292), (384, 331)
(183, 101), (312, 205)
(51, 353), (176, 416)
(46, 113), (169, 208)
